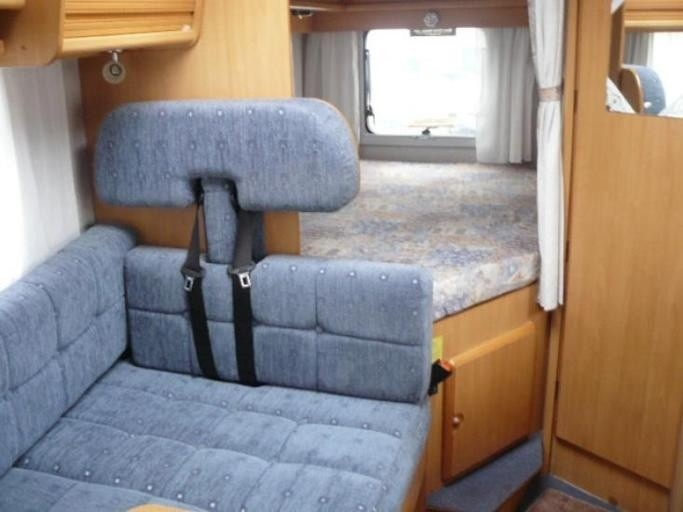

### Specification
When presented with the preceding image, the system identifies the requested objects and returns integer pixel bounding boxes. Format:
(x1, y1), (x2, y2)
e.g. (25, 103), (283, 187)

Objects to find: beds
(301, 159), (552, 499)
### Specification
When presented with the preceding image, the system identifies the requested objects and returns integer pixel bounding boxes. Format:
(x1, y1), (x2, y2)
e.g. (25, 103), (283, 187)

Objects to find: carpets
(507, 475), (623, 512)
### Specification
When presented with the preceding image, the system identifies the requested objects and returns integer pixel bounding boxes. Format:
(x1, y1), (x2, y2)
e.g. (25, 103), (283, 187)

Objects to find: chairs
(0, 99), (433, 511)
